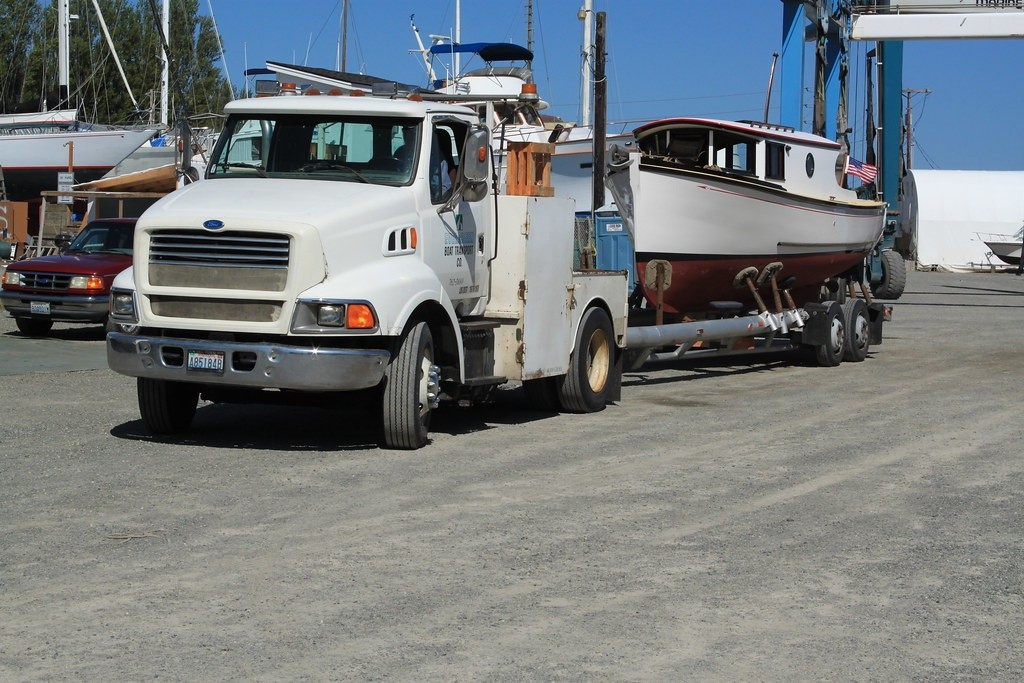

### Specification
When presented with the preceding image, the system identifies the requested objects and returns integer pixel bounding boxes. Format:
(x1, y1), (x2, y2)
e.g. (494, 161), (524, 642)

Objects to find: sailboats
(0, 0), (556, 235)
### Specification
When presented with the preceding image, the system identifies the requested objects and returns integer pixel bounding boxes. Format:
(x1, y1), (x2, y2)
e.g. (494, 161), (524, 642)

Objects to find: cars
(0, 217), (139, 337)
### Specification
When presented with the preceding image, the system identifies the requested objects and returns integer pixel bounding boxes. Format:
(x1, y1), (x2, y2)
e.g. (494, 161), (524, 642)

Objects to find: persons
(393, 127), (452, 201)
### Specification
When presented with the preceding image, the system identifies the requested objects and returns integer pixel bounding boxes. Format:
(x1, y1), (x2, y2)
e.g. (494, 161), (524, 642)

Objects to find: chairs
(436, 129), (456, 189)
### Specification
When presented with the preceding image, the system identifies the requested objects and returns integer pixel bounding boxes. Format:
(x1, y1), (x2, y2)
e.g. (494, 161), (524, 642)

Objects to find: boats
(975, 219), (1024, 266)
(604, 51), (890, 351)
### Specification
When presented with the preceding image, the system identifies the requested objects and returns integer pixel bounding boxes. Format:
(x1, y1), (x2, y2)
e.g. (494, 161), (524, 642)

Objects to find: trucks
(104, 0), (893, 449)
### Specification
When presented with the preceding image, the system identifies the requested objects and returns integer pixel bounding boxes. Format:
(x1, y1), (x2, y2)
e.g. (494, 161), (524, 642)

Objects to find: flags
(845, 155), (878, 186)
